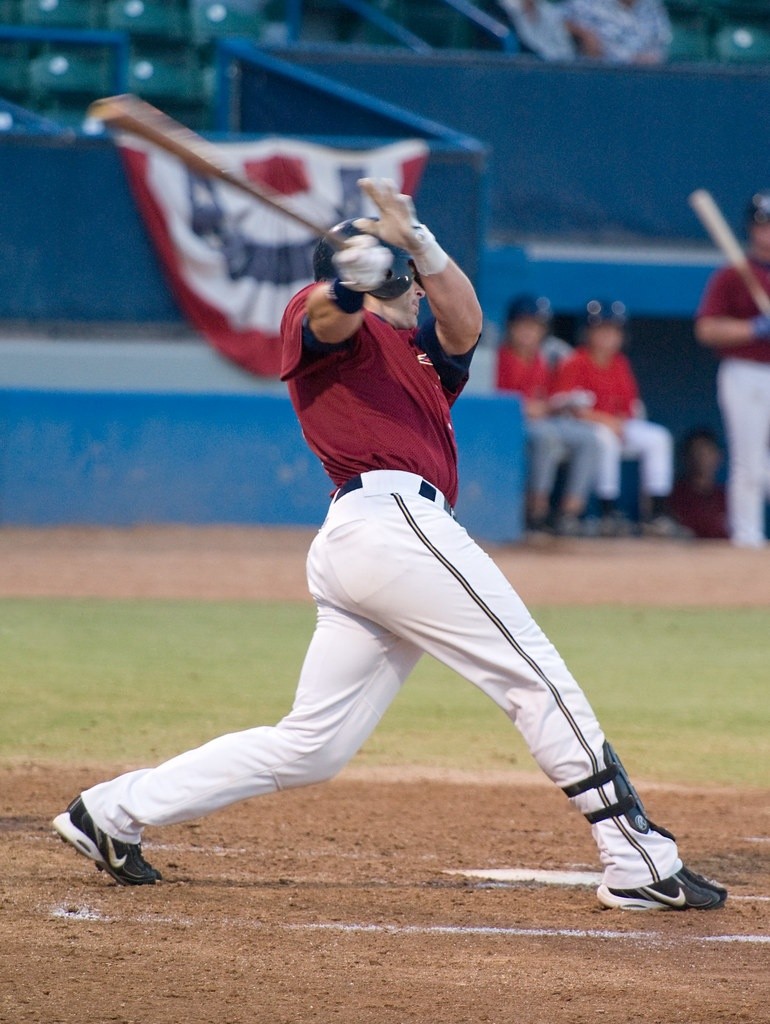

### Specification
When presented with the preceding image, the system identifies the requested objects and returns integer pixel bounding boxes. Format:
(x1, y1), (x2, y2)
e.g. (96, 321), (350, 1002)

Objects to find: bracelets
(411, 242), (450, 278)
(326, 278), (365, 314)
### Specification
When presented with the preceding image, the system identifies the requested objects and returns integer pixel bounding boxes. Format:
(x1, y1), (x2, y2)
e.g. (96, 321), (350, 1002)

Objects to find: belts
(335, 472), (459, 523)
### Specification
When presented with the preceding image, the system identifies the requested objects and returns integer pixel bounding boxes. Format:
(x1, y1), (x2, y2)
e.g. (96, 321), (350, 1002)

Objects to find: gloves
(329, 237), (391, 290)
(752, 314), (770, 339)
(353, 172), (448, 276)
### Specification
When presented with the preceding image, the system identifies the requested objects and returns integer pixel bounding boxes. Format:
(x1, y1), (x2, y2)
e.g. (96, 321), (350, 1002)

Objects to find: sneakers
(53, 796), (163, 887)
(595, 866), (726, 913)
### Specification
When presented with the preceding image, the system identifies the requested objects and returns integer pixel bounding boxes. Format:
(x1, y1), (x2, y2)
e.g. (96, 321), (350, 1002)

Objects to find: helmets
(314, 217), (418, 299)
(587, 300), (625, 329)
(746, 192), (769, 225)
(508, 293), (550, 322)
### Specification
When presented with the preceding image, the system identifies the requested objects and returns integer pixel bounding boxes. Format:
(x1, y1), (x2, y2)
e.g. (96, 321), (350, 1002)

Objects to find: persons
(557, 298), (695, 538)
(51, 175), (728, 912)
(667, 425), (728, 538)
(495, 292), (599, 537)
(505, 0), (598, 63)
(570, 0), (677, 66)
(701, 192), (770, 550)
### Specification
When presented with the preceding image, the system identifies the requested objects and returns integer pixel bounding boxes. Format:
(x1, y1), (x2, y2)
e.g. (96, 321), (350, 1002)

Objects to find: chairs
(0, 0), (769, 128)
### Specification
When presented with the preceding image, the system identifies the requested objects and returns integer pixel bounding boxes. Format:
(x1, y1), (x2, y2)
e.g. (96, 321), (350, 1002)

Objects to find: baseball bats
(85, 91), (393, 280)
(687, 188), (770, 318)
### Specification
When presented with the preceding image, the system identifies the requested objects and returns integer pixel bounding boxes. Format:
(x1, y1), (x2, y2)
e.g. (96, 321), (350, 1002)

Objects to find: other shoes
(529, 509), (590, 538)
(600, 510), (636, 538)
(642, 512), (681, 538)
